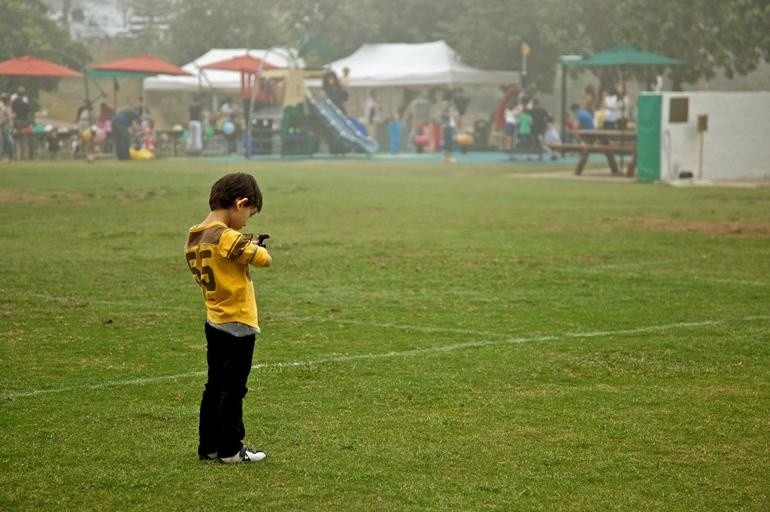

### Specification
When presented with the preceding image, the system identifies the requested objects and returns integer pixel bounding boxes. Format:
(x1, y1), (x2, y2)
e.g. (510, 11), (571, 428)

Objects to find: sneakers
(199, 451), (217, 459)
(217, 447), (266, 464)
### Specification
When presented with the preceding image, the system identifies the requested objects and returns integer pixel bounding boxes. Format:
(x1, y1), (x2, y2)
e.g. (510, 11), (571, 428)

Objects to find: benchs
(549, 129), (637, 176)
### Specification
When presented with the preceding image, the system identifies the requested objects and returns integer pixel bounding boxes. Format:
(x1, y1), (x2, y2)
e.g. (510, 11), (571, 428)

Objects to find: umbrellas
(90, 66), (158, 114)
(560, 47), (685, 178)
(0, 53), (83, 108)
(191, 56), (283, 96)
(93, 54), (200, 116)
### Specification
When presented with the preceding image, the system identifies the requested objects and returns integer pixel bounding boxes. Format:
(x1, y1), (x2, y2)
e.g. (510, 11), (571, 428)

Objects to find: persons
(183, 172), (273, 463)
(339, 68), (350, 89)
(0, 81), (277, 164)
(324, 82), (641, 165)
